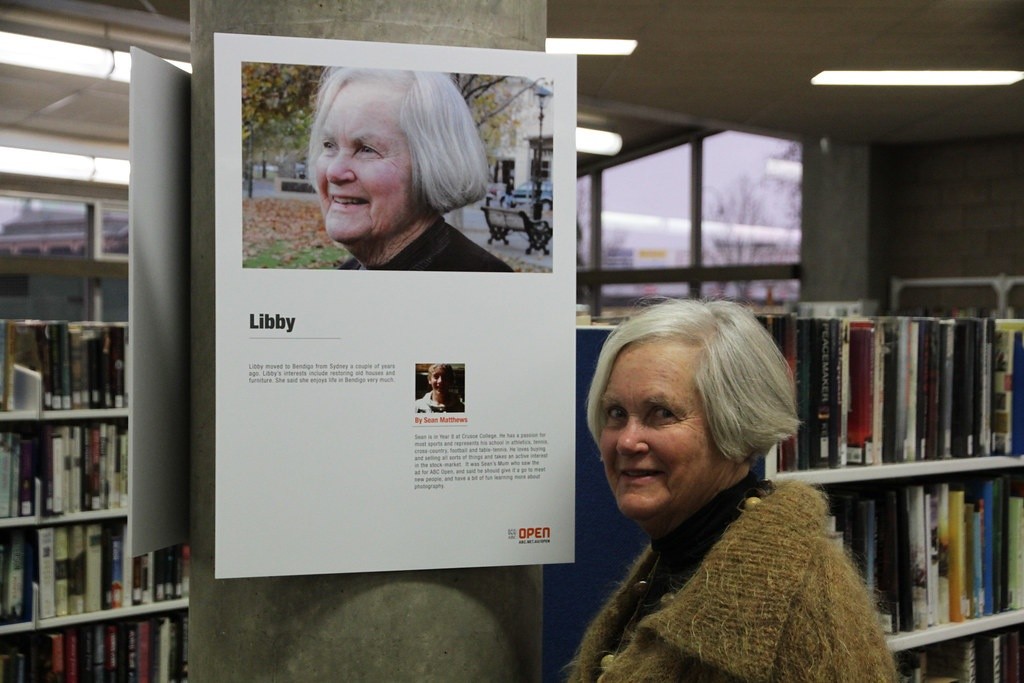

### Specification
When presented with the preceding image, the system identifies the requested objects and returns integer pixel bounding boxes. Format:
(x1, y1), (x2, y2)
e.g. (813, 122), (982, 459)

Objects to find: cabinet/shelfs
(0, 293), (1024, 682)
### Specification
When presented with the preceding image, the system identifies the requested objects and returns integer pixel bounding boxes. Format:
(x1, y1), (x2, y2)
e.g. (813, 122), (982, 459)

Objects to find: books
(740, 300), (1024, 683)
(0, 322), (191, 683)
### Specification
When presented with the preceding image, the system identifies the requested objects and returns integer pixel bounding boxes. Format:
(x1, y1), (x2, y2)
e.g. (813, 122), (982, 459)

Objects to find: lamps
(0, 0), (207, 96)
(576, 125), (622, 157)
(0, 132), (130, 202)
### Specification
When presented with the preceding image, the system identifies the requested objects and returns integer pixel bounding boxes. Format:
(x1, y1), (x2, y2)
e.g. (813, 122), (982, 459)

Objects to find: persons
(565, 298), (899, 683)
(415, 363), (464, 413)
(306, 65), (514, 273)
